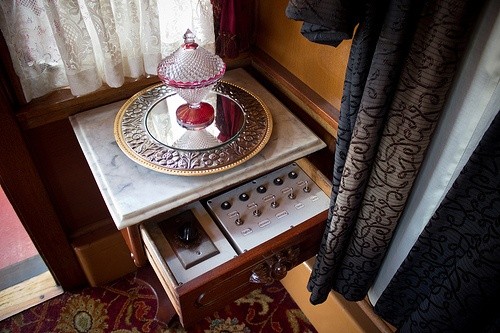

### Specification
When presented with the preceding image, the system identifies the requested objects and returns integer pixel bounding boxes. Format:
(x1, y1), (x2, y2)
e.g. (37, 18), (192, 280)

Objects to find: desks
(68, 66), (342, 328)
